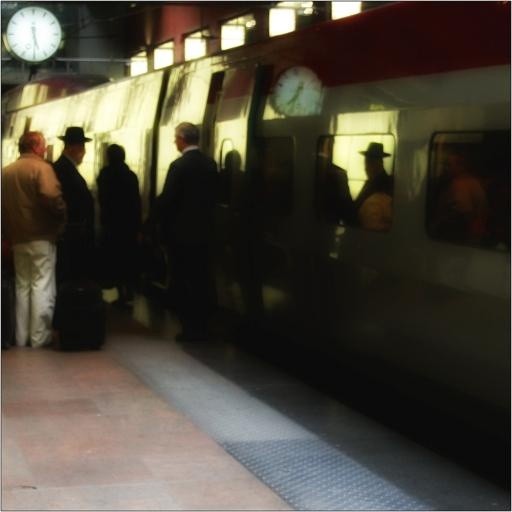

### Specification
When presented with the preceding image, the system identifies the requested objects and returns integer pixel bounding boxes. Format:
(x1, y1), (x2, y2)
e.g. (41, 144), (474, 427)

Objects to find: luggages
(53, 237), (105, 351)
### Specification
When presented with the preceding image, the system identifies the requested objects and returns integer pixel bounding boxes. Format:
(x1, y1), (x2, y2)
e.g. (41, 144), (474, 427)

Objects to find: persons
(146, 121), (217, 344)
(1, 131), (68, 348)
(318, 135), (354, 219)
(433, 137), (489, 244)
(355, 142), (392, 211)
(229, 142), (269, 348)
(217, 150), (245, 202)
(51, 126), (95, 283)
(95, 143), (142, 308)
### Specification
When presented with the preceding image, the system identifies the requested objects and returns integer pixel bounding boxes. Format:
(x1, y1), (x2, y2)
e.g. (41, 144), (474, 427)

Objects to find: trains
(0, 0), (509, 499)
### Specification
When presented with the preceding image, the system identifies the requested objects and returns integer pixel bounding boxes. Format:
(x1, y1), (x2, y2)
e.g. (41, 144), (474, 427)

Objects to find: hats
(359, 143), (389, 157)
(58, 128), (93, 142)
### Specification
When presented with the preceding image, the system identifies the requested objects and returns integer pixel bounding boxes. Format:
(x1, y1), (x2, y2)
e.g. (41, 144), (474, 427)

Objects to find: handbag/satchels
(127, 239), (169, 290)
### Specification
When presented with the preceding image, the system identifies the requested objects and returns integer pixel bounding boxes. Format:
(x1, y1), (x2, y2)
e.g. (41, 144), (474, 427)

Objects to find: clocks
(270, 64), (325, 121)
(3, 3), (66, 67)
